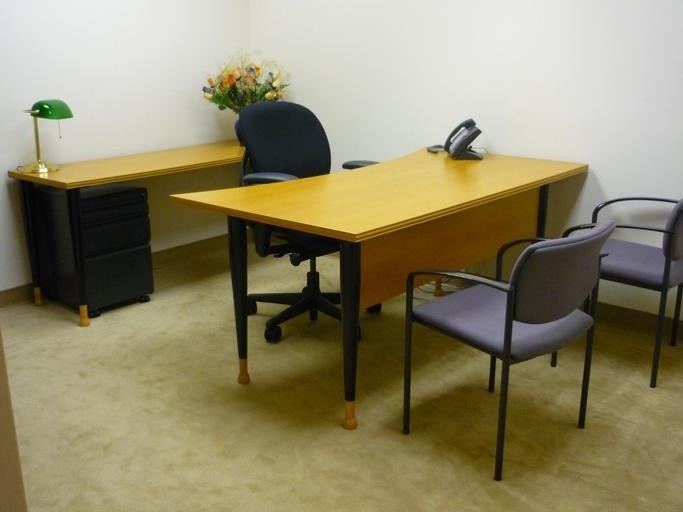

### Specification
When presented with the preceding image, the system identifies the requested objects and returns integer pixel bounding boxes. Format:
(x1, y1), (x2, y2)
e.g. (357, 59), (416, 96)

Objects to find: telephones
(444, 118), (483, 160)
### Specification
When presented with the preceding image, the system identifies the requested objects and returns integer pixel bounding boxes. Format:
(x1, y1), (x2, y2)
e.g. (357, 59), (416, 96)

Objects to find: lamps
(16, 100), (74, 173)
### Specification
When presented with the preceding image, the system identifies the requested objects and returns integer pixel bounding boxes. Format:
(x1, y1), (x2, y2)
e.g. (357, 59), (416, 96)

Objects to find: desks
(8, 140), (246, 326)
(168, 146), (589, 430)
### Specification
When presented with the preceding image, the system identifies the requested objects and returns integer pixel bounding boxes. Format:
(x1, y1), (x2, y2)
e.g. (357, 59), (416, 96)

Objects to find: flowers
(202, 63), (291, 114)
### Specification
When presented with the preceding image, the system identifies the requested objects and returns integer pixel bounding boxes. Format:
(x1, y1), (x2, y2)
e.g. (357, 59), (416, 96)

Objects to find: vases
(235, 113), (245, 146)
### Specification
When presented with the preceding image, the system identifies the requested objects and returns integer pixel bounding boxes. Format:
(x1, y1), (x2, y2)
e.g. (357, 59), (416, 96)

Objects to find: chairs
(234, 101), (381, 343)
(551, 198), (683, 388)
(403, 220), (616, 481)
